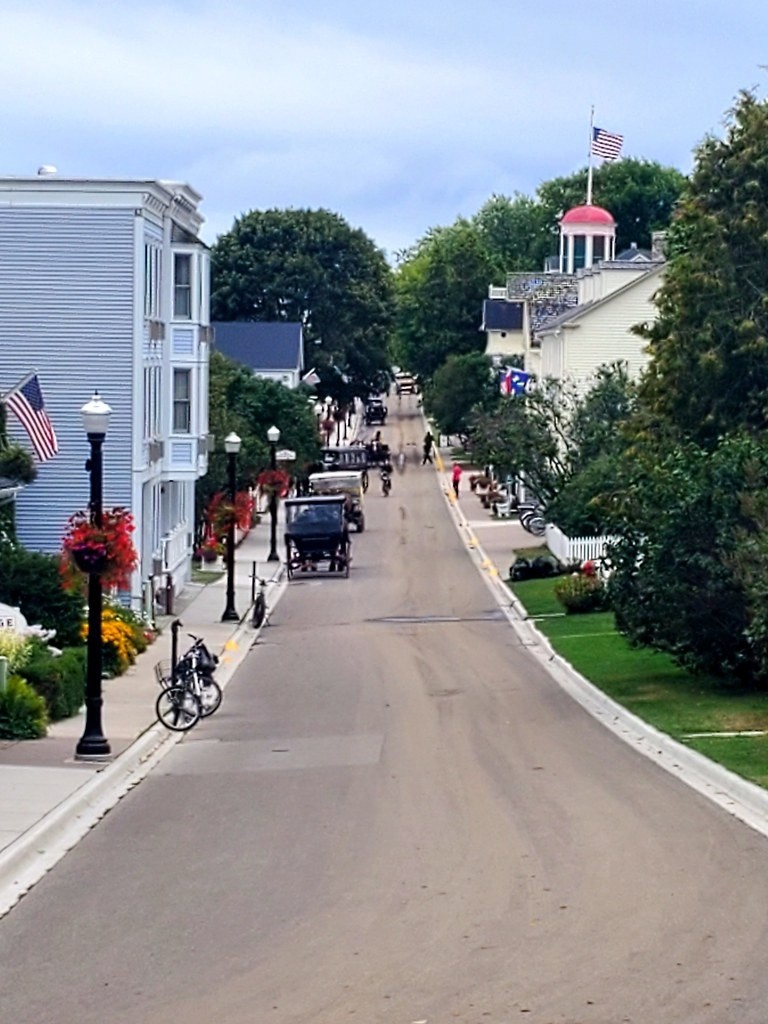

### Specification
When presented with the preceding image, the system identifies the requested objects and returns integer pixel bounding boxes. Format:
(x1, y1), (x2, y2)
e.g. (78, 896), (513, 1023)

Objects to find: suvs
(395, 373), (415, 394)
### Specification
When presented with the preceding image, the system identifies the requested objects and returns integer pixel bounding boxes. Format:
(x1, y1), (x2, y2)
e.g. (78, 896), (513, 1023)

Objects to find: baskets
(155, 659), (173, 682)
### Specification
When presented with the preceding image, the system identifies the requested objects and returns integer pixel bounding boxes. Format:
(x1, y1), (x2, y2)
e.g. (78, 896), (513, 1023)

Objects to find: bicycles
(381, 474), (390, 495)
(154, 634), (222, 731)
(250, 575), (278, 627)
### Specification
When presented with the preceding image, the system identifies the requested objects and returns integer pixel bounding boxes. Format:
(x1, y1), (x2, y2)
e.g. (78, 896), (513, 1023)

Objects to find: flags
(500, 370), (528, 399)
(5, 374), (60, 463)
(591, 126), (624, 159)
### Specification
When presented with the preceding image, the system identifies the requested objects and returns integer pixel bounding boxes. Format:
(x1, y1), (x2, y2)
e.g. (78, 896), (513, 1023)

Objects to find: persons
(452, 462), (462, 499)
(422, 431), (435, 465)
(380, 457), (393, 491)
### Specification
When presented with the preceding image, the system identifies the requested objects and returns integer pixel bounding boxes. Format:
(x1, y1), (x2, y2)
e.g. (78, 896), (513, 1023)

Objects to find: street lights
(325, 395), (333, 446)
(75, 390), (111, 762)
(315, 402), (323, 432)
(266, 426), (281, 562)
(219, 431), (241, 621)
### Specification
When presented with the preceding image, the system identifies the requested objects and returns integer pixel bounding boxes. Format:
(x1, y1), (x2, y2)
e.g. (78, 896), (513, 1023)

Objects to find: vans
(321, 448), (368, 489)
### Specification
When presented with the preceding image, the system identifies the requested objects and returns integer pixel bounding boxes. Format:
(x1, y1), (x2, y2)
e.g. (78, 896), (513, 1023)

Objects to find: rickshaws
(308, 471), (364, 532)
(363, 399), (388, 426)
(363, 431), (391, 467)
(285, 497), (351, 579)
